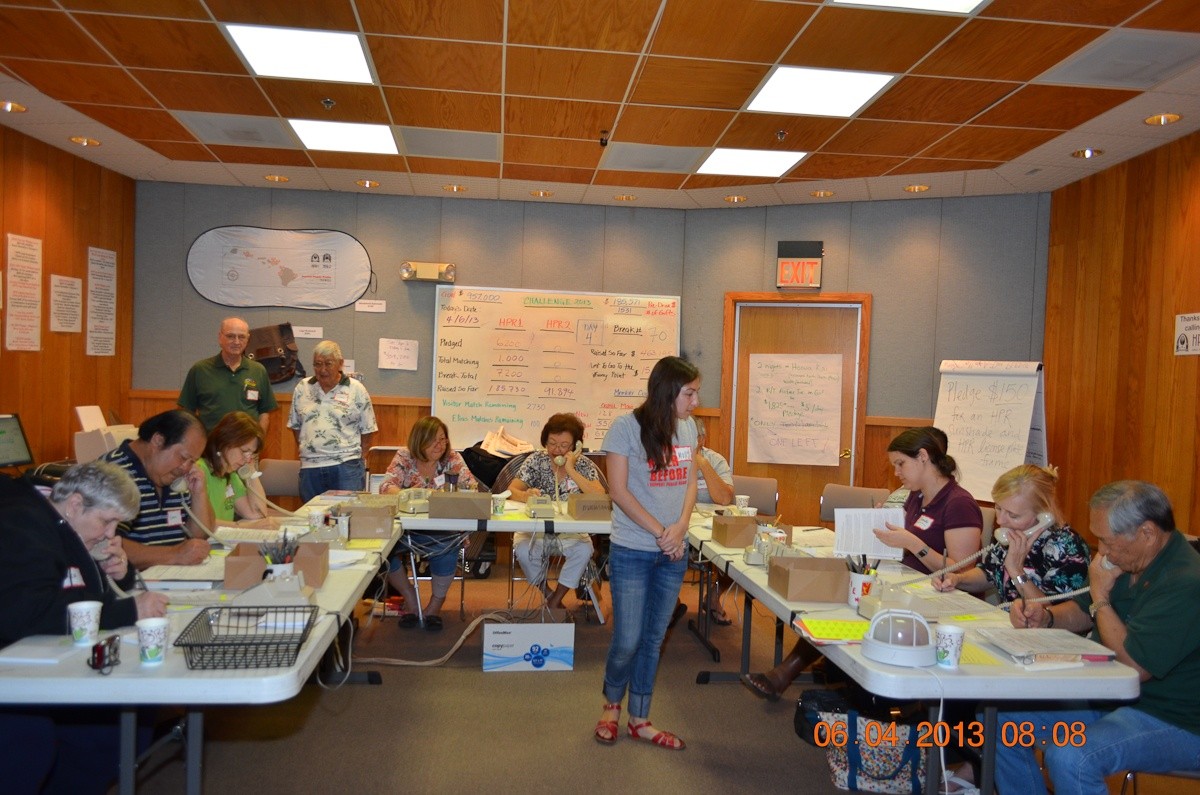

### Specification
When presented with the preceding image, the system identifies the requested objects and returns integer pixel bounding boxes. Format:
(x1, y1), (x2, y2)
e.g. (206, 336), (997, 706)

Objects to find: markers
(593, 450), (602, 452)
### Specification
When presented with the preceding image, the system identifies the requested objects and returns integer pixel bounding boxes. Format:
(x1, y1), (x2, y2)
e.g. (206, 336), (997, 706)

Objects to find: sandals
(939, 776), (980, 795)
(594, 704), (622, 745)
(626, 721), (685, 750)
(940, 770), (953, 782)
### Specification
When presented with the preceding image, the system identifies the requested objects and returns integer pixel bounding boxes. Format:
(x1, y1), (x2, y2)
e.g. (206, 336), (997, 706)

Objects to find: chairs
(257, 459), (1002, 626)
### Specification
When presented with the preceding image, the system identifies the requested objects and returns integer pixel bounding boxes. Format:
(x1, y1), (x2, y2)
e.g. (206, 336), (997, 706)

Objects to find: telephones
(88, 538), (113, 560)
(237, 463), (254, 480)
(170, 477), (188, 494)
(554, 440), (583, 467)
(1101, 553), (1118, 571)
(994, 512), (1056, 546)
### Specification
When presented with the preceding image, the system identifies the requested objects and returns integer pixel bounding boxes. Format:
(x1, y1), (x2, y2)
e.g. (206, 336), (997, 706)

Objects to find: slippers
(740, 672), (781, 703)
(702, 602), (733, 626)
(668, 603), (687, 628)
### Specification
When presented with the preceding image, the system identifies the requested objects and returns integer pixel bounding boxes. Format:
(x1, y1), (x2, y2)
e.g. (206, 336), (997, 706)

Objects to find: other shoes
(398, 614), (419, 629)
(423, 615), (443, 631)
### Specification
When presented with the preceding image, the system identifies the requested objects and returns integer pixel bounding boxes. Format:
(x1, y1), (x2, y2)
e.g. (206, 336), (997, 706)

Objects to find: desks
(0, 491), (1140, 795)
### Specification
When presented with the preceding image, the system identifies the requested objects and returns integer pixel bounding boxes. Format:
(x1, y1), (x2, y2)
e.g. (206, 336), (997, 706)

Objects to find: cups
(135, 617), (168, 667)
(741, 506), (757, 518)
(68, 601), (103, 644)
(492, 494), (504, 514)
(329, 515), (350, 541)
(736, 494), (748, 508)
(935, 624), (964, 670)
(849, 570), (877, 608)
(310, 511), (324, 535)
(266, 564), (294, 579)
(769, 532), (787, 544)
(757, 525), (784, 535)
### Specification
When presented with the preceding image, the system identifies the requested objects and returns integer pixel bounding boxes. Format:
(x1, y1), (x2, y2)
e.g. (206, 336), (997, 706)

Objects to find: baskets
(173, 605), (319, 669)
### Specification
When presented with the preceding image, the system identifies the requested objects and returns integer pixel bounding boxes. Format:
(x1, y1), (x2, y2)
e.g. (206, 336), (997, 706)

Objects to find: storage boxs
(768, 556), (849, 602)
(428, 492), (491, 520)
(480, 609), (576, 672)
(331, 494), (399, 539)
(224, 541), (330, 589)
(712, 516), (757, 548)
(567, 494), (611, 521)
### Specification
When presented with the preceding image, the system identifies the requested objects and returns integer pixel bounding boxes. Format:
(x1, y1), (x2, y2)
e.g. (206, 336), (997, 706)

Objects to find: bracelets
(915, 545), (928, 559)
(656, 528), (665, 539)
(1046, 608), (1054, 628)
(250, 471), (262, 479)
(1011, 575), (1030, 585)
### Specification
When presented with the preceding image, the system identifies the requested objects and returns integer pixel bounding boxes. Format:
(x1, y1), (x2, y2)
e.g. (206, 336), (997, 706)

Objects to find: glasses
(239, 447), (258, 459)
(313, 360), (337, 367)
(546, 439), (573, 452)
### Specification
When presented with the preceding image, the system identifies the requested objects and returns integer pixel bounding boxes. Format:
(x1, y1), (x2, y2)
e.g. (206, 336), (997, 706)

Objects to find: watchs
(1089, 601), (1111, 617)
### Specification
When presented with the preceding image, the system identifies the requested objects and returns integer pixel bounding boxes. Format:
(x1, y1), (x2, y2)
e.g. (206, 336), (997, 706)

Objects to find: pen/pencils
(425, 477), (429, 488)
(257, 527), (301, 565)
(802, 527), (825, 531)
(754, 516), (772, 527)
(939, 548), (948, 594)
(330, 505), (352, 517)
(846, 552), (881, 576)
(774, 514), (782, 527)
(1020, 587), (1029, 628)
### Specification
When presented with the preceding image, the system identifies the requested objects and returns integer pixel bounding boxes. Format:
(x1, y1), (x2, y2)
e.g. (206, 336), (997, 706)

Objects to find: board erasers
(582, 447), (589, 452)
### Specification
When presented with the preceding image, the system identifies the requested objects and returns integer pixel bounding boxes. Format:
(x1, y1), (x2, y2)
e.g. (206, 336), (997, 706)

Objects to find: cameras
(92, 635), (120, 669)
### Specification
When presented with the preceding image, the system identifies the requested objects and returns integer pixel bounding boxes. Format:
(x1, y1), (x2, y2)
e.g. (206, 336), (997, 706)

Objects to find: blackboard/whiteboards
(932, 360), (1042, 503)
(429, 284), (682, 456)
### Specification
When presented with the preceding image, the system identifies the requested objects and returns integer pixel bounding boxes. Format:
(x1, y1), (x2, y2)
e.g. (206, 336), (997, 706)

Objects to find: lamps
(399, 261), (455, 282)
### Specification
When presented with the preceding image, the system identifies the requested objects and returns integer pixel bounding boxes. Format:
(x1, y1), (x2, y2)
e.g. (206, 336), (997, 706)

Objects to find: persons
(595, 356), (701, 751)
(173, 318), (279, 471)
(285, 341), (378, 504)
(931, 463), (1093, 794)
(668, 416), (736, 628)
(507, 412), (606, 622)
(95, 408), (213, 573)
(378, 416), (478, 631)
(972, 480), (1200, 795)
(194, 410), (281, 536)
(741, 427), (984, 701)
(0, 460), (168, 795)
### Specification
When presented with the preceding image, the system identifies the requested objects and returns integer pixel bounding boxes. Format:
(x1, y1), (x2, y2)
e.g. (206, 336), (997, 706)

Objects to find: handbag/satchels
(820, 710), (927, 795)
(794, 689), (860, 747)
(241, 322), (305, 384)
